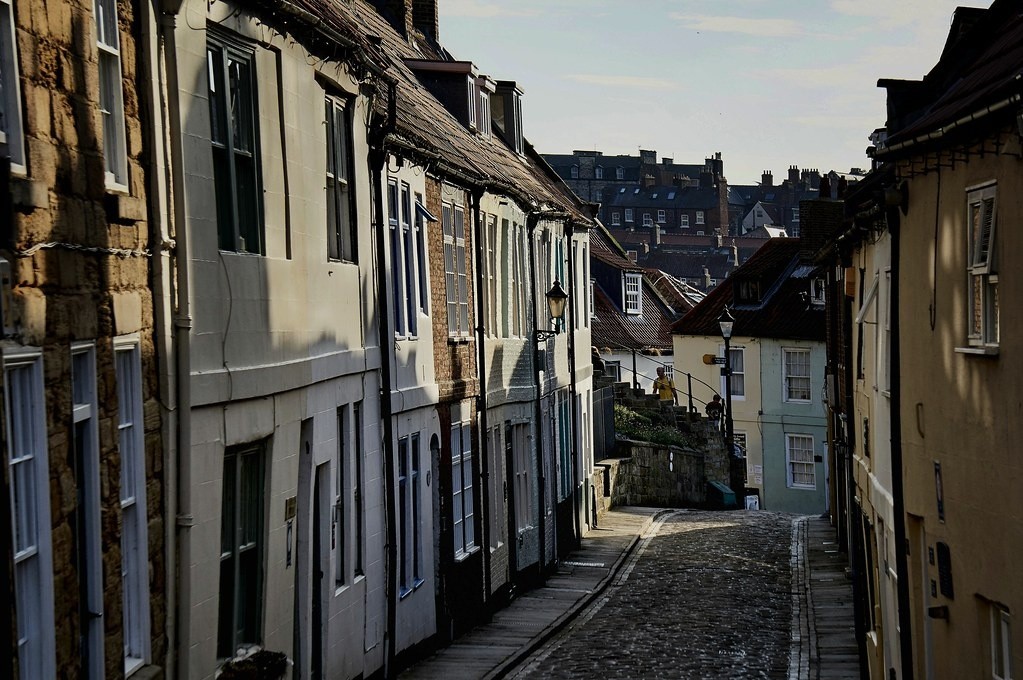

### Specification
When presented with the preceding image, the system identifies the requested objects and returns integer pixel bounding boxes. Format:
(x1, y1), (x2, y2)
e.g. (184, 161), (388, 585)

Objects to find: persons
(705, 395), (725, 431)
(801, 391), (806, 399)
(653, 367), (679, 407)
(749, 501), (757, 510)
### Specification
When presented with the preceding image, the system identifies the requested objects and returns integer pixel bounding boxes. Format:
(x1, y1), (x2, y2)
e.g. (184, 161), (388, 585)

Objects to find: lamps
(534, 274), (570, 345)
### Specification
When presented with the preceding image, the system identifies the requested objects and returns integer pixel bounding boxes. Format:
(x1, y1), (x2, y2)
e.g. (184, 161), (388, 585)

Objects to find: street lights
(718, 304), (738, 488)
